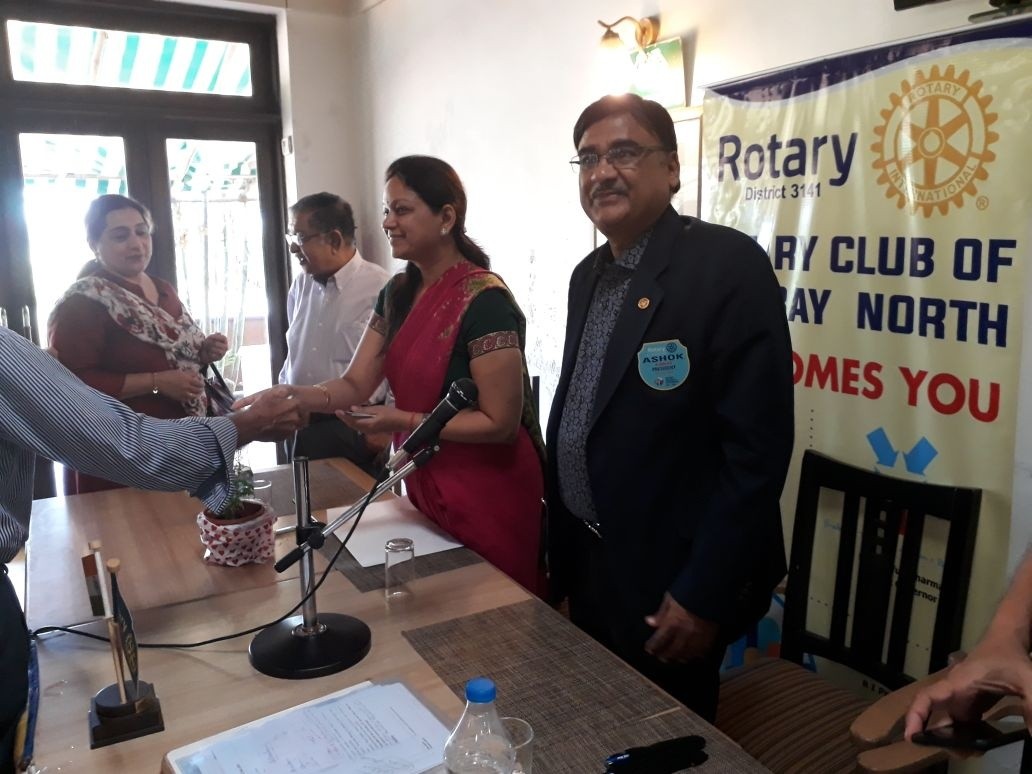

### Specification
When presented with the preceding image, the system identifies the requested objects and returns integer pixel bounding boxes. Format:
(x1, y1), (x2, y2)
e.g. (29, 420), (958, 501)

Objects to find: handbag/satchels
(204, 362), (236, 417)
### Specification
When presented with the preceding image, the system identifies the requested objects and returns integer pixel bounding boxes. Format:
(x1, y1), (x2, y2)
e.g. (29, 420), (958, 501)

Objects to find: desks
(23, 458), (770, 774)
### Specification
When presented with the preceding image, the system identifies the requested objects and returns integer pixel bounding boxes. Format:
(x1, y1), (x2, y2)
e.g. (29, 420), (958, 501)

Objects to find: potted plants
(202, 461), (265, 526)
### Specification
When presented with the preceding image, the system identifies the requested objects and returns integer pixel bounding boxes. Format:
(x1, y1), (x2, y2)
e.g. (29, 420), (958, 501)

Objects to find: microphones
(383, 377), (478, 472)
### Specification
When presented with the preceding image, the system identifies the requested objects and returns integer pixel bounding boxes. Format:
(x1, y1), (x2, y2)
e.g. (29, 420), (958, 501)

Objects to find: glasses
(284, 230), (330, 246)
(569, 146), (671, 174)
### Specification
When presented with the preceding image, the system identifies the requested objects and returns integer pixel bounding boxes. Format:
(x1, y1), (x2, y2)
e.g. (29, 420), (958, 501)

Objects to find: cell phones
(910, 719), (1029, 751)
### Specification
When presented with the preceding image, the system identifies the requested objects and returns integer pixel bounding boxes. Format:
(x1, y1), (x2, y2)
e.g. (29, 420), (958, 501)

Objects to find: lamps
(597, 16), (660, 48)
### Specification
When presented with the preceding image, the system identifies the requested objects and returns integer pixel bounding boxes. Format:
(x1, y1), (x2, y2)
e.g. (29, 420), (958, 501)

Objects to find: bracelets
(312, 382), (331, 414)
(151, 371), (160, 396)
(407, 408), (419, 432)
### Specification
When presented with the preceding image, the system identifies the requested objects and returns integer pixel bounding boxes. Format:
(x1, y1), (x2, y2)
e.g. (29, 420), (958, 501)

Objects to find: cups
(252, 479), (274, 506)
(499, 716), (534, 774)
(384, 537), (416, 604)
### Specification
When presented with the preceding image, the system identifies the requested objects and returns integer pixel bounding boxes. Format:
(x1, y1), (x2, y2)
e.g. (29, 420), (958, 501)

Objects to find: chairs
(699, 450), (983, 774)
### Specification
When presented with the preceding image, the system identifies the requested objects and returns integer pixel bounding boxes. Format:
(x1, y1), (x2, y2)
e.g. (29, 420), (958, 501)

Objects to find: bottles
(441, 677), (519, 774)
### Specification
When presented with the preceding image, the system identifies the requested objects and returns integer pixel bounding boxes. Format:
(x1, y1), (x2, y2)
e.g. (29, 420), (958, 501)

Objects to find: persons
(274, 192), (391, 463)
(545, 95), (795, 717)
(903, 542), (1032, 742)
(231, 154), (543, 599)
(0, 323), (300, 773)
(44, 193), (230, 496)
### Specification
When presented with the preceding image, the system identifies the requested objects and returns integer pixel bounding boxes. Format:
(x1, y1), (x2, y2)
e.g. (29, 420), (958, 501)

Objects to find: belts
(583, 519), (608, 540)
